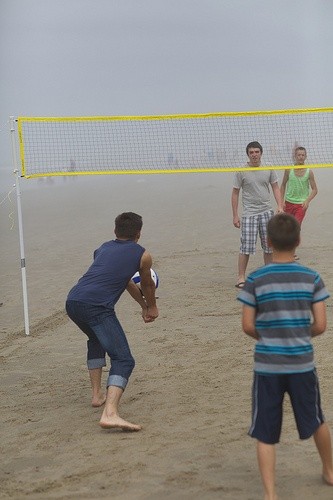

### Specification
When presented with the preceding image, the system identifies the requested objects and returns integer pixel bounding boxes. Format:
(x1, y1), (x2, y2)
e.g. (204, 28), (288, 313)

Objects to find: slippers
(234, 282), (245, 289)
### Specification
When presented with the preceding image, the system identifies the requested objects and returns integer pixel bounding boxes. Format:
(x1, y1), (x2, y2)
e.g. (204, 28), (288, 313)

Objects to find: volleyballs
(132, 267), (160, 293)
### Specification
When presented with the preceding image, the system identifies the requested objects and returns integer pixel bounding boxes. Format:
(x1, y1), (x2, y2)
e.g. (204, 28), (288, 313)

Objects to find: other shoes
(294, 255), (299, 260)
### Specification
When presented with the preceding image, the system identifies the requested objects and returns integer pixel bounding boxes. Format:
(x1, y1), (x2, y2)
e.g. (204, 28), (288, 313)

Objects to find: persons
(280, 147), (318, 257)
(236, 213), (333, 500)
(66, 212), (159, 431)
(232, 142), (286, 288)
